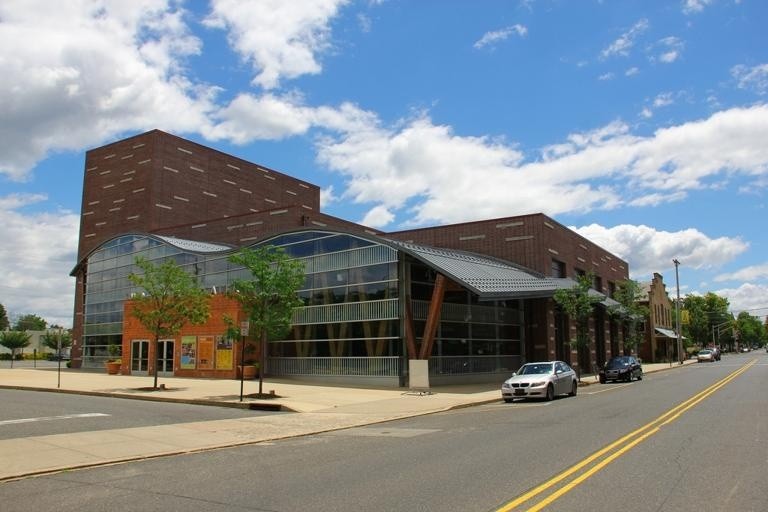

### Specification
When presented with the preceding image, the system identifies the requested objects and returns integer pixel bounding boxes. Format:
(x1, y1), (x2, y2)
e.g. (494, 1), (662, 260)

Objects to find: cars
(598, 356), (644, 386)
(500, 361), (578, 403)
(697, 347), (721, 363)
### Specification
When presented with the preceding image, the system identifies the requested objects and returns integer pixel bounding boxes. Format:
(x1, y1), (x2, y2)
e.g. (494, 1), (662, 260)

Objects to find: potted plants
(237, 344), (262, 379)
(105, 345), (121, 374)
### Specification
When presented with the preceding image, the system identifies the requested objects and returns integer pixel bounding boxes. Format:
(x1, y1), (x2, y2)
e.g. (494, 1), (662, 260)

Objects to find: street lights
(673, 258), (684, 364)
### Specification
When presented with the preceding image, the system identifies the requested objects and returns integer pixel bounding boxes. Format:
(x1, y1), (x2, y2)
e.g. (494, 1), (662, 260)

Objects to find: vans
(60, 346), (72, 360)
